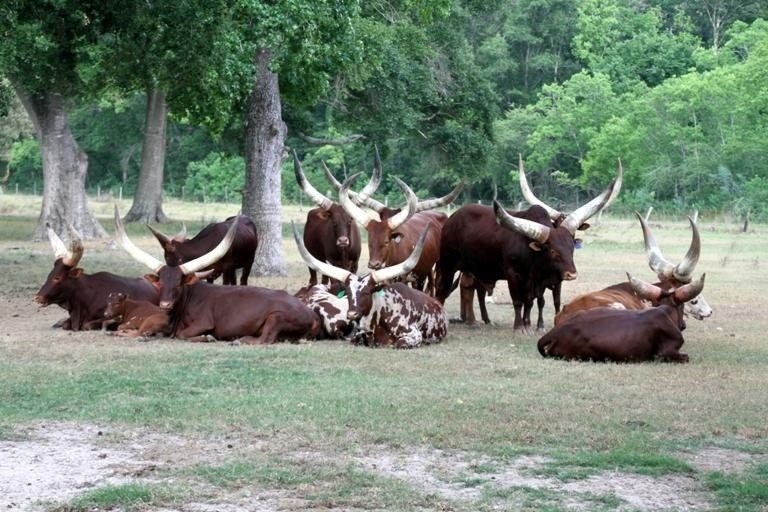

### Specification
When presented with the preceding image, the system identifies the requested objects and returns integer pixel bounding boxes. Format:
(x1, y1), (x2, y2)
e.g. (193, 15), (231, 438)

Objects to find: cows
(146, 213), (258, 287)
(553, 209), (713, 330)
(82, 290), (174, 343)
(32, 217), (160, 331)
(536, 273), (707, 364)
(287, 145), (624, 348)
(114, 202), (321, 348)
